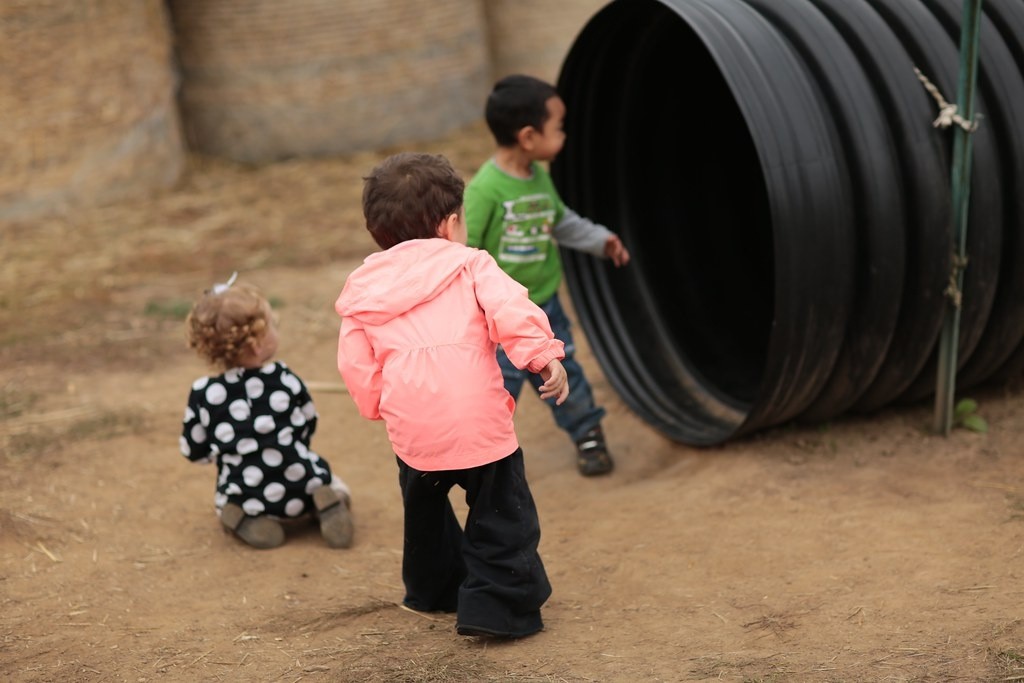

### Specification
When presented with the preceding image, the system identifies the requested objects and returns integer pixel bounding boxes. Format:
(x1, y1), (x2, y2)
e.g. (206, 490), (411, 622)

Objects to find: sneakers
(576, 425), (613, 478)
(313, 485), (354, 548)
(221, 506), (285, 549)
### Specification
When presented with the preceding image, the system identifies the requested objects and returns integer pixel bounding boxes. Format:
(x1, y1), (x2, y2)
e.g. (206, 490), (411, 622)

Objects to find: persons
(335, 154), (570, 642)
(461, 74), (629, 480)
(179, 270), (354, 550)
